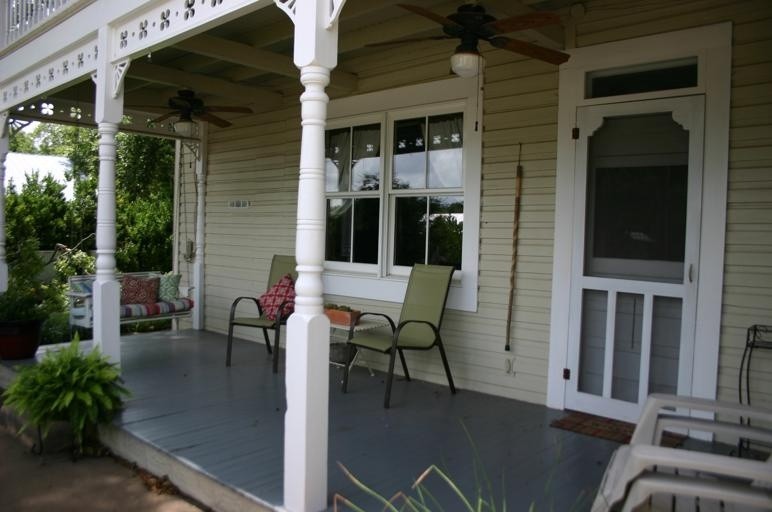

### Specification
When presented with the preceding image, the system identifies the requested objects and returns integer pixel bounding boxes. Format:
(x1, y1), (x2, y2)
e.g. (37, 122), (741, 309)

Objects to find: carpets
(551, 412), (687, 451)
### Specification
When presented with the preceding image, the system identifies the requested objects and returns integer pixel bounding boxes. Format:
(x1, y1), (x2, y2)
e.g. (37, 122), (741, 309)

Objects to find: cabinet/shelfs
(737, 324), (772, 462)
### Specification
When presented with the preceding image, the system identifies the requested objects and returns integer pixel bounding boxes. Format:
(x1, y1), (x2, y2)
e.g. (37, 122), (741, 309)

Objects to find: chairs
(587, 392), (772, 512)
(342, 263), (457, 410)
(226, 254), (298, 373)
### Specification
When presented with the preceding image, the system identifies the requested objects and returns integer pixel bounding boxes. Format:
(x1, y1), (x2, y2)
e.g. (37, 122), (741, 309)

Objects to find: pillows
(149, 272), (182, 303)
(120, 274), (160, 304)
(257, 272), (295, 321)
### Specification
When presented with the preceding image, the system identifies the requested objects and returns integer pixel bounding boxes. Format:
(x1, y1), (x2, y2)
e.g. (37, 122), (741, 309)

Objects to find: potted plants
(324, 302), (361, 326)
(1, 332), (133, 465)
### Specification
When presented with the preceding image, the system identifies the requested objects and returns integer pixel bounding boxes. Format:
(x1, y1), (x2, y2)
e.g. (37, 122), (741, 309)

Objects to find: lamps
(449, 33), (487, 80)
(174, 114), (198, 137)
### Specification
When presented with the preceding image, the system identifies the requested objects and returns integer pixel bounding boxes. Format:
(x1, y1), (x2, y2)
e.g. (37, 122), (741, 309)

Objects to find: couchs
(63, 271), (194, 346)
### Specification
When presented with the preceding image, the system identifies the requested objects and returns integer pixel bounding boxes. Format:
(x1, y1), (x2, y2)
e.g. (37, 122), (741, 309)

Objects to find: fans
(128, 89), (254, 128)
(363, 3), (570, 74)
